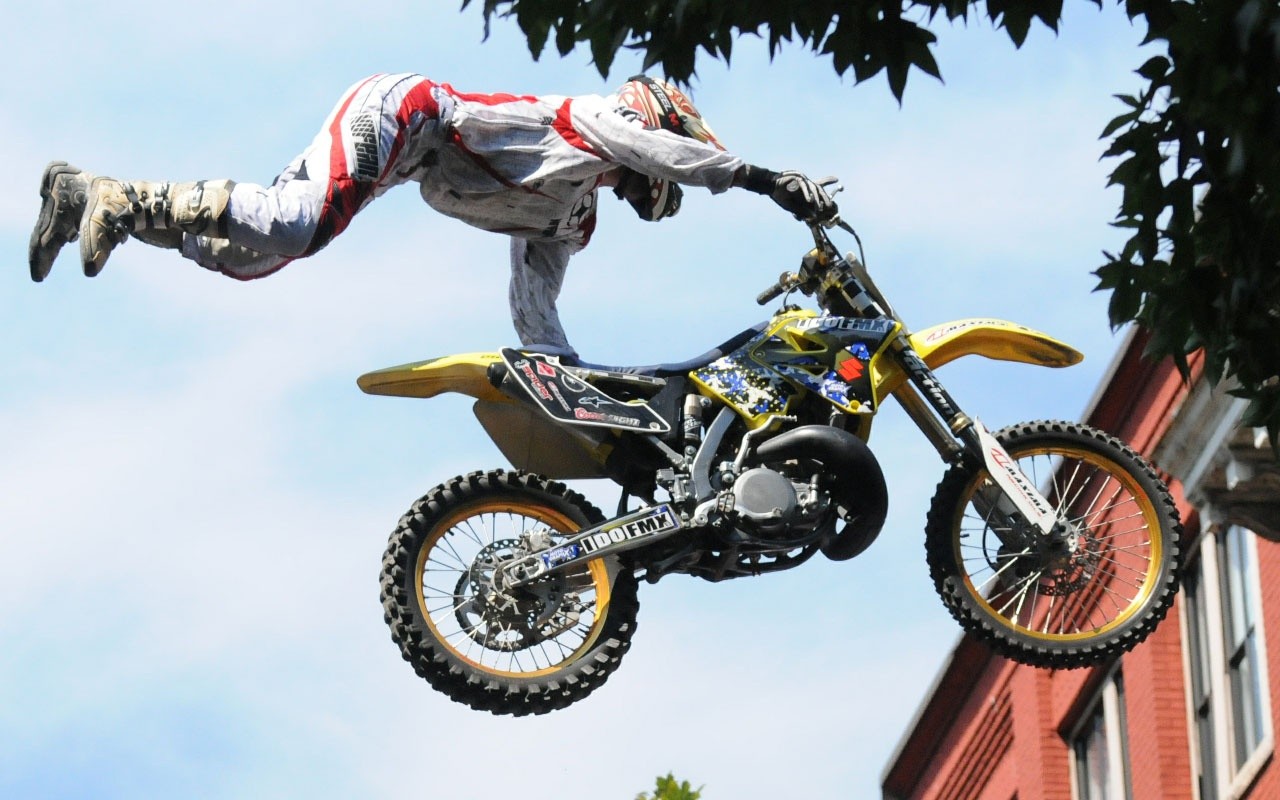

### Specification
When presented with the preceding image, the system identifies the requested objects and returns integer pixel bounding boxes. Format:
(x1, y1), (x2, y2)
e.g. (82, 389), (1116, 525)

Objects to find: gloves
(744, 162), (837, 217)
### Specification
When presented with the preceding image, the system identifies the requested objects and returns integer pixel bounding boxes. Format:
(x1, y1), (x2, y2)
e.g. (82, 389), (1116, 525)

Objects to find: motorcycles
(354, 178), (1192, 720)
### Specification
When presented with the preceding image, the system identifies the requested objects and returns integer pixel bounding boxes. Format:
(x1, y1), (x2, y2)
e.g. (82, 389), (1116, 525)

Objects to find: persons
(29, 74), (837, 361)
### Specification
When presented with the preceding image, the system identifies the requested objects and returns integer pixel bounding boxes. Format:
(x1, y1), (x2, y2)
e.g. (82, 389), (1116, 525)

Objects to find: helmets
(614, 76), (728, 222)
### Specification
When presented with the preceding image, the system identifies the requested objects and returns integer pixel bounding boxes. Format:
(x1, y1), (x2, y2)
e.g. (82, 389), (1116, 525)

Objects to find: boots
(28, 162), (184, 283)
(80, 176), (238, 277)
